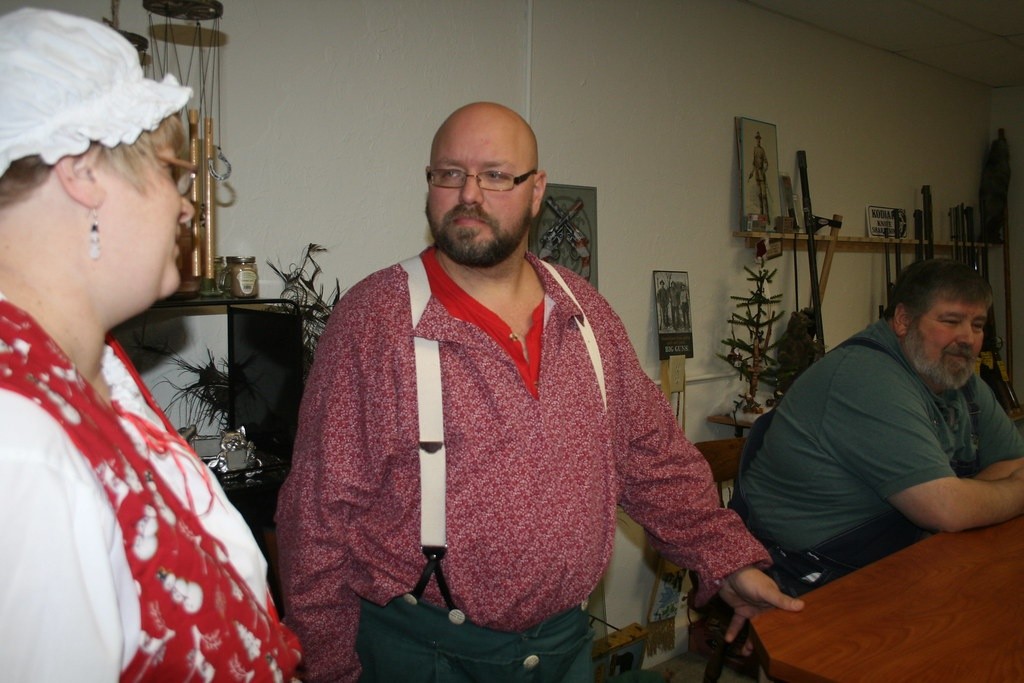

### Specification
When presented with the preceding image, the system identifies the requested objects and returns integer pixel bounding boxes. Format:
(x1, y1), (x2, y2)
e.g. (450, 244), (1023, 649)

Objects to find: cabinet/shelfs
(150, 292), (304, 554)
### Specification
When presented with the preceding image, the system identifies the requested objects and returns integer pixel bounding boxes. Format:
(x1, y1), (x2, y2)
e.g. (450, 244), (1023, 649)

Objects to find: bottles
(212, 255), (259, 298)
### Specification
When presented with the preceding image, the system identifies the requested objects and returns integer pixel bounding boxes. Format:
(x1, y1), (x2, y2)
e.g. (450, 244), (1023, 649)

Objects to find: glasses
(155, 152), (197, 195)
(430, 169), (537, 191)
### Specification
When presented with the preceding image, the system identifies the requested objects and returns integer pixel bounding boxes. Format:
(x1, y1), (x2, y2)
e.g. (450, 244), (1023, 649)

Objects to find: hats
(0, 7), (193, 175)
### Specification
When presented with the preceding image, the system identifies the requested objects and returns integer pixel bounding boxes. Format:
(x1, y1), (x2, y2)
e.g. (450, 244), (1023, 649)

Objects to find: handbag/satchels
(799, 510), (937, 573)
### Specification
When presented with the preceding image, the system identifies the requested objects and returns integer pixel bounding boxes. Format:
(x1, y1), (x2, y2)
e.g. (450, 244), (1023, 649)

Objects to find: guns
(883, 183), (934, 308)
(949, 199), (1020, 415)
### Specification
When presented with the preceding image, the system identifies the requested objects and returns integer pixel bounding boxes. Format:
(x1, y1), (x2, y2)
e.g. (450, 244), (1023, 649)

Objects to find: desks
(706, 414), (753, 434)
(754, 515), (1024, 683)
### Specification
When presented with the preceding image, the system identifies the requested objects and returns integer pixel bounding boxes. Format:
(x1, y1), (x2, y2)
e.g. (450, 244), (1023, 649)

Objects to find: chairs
(689, 438), (759, 683)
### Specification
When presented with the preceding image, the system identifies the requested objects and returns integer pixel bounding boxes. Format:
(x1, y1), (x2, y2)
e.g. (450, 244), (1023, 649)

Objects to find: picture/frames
(735, 115), (780, 231)
(529, 183), (599, 293)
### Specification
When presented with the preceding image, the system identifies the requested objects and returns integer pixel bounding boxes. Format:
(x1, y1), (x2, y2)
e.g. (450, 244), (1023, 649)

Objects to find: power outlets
(669, 354), (685, 393)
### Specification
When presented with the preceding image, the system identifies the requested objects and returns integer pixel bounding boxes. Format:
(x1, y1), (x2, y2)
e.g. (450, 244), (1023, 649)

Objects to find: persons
(277, 102), (805, 683)
(726, 259), (1024, 643)
(749, 132), (771, 225)
(657, 280), (691, 328)
(0, 10), (306, 682)
